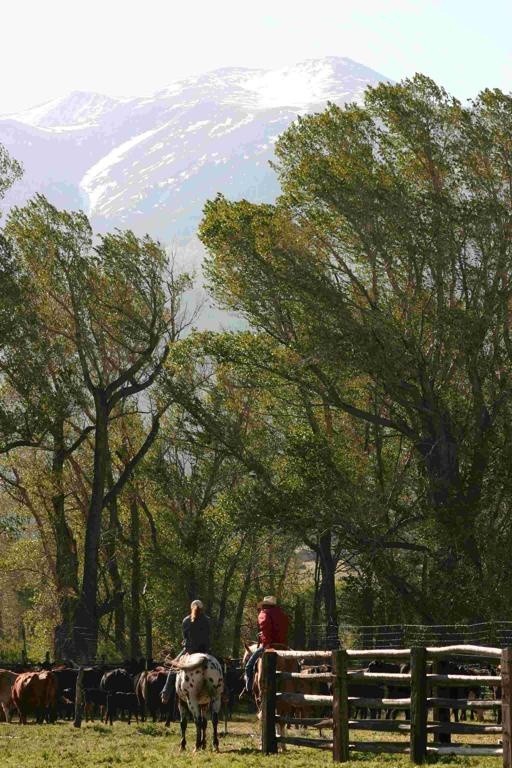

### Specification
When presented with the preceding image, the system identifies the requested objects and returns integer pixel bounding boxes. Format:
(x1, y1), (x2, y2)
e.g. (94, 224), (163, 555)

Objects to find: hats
(190, 599), (203, 609)
(262, 595), (277, 605)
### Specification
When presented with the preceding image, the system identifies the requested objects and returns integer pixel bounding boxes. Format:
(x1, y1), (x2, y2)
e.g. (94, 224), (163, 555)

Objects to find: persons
(237, 593), (303, 702)
(158, 598), (215, 706)
(494, 663), (502, 724)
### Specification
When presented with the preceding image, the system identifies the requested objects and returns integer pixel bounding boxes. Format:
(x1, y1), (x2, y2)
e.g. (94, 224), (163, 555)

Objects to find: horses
(164, 652), (224, 756)
(242, 637), (300, 753)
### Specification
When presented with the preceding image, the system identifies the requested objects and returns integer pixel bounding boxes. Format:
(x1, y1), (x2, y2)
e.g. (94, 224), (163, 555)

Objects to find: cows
(286, 657), (502, 731)
(0, 667), (174, 725)
